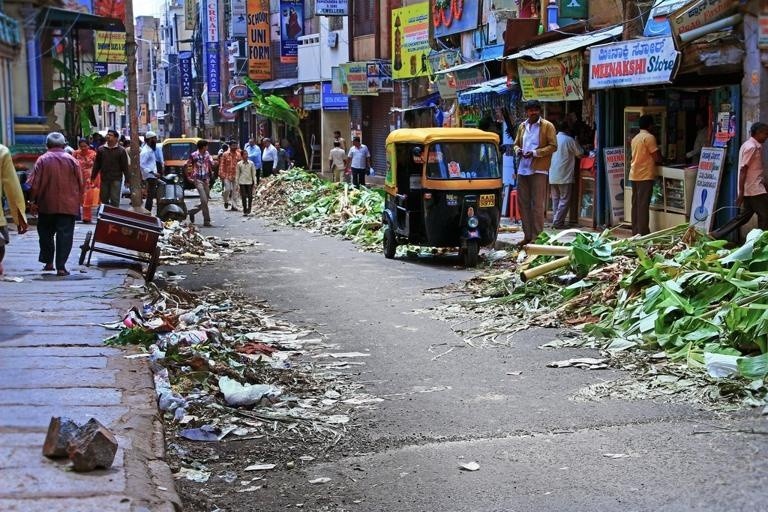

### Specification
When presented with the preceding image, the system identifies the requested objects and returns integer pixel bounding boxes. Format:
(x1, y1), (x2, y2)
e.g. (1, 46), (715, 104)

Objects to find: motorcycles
(202, 139), (225, 169)
(159, 132), (204, 185)
(14, 170), (32, 200)
(157, 172), (188, 223)
(2, 170), (34, 217)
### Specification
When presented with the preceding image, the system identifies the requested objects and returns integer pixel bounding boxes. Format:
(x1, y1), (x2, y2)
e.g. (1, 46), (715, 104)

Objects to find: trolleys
(77, 200), (163, 286)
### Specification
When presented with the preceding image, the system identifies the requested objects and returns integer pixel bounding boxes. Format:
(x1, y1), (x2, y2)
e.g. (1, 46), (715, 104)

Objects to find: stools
(507, 188), (524, 225)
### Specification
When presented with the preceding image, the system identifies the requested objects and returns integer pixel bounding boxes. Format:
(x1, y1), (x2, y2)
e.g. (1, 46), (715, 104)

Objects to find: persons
(708, 121), (768, 239)
(334, 131), (346, 150)
(629, 115), (663, 236)
(347, 137), (371, 189)
(548, 121), (584, 229)
(557, 112), (589, 143)
(0, 144), (29, 274)
(63, 129), (296, 226)
(26, 132), (84, 276)
(513, 100), (557, 245)
(328, 141), (348, 182)
(685, 112), (708, 166)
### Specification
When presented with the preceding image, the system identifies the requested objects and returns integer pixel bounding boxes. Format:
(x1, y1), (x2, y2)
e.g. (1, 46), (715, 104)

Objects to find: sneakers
(187, 209), (194, 223)
(82, 219), (93, 224)
(41, 263), (55, 270)
(203, 220), (213, 227)
(57, 268), (68, 276)
(242, 212), (254, 217)
(222, 201), (239, 211)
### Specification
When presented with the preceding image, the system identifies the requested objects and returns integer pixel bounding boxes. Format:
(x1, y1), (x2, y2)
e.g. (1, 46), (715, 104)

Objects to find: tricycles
(380, 124), (503, 265)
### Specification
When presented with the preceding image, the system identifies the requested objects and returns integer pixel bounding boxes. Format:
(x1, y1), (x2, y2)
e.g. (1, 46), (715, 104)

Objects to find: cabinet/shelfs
(624, 105), (667, 229)
(646, 161), (699, 215)
(544, 177), (579, 223)
(576, 173), (596, 222)
